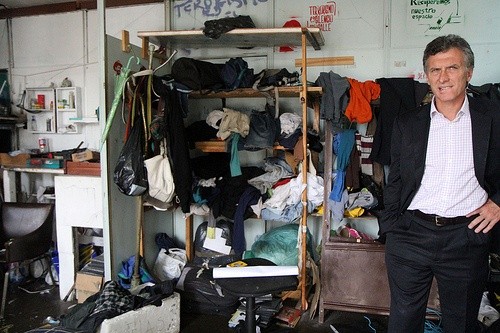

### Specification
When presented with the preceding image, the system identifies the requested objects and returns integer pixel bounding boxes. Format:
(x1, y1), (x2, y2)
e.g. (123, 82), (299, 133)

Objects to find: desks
(1, 166), (65, 199)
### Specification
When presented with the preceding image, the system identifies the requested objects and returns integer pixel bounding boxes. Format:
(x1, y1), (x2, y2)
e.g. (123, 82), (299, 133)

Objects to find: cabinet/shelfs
(135, 25), (327, 315)
(25, 87), (55, 134)
(56, 87), (81, 133)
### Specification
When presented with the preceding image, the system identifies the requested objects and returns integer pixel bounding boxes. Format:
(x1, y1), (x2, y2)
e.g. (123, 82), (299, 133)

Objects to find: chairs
(0, 202), (56, 317)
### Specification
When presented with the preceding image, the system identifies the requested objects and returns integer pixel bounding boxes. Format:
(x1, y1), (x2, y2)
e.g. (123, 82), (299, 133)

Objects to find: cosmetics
(58, 91), (73, 108)
(31, 116), (53, 131)
(30, 95), (53, 109)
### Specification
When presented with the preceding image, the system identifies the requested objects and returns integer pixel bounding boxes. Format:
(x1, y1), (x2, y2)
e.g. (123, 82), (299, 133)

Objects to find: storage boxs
(97, 292), (181, 333)
(0, 147), (100, 177)
(76, 257), (107, 304)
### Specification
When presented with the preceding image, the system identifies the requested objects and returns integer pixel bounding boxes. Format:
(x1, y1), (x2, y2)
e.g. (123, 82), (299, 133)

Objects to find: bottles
(31, 117), (37, 131)
(39, 138), (45, 153)
(50, 101), (53, 110)
(69, 91), (75, 109)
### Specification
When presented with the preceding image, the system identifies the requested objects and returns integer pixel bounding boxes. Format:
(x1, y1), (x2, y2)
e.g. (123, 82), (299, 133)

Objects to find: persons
(378, 34), (500, 333)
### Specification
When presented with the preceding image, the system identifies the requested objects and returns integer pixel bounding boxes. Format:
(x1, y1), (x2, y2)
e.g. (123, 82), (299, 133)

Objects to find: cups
(38, 94), (45, 109)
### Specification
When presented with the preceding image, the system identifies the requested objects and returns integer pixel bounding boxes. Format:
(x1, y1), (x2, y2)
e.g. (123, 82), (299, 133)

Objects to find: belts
(407, 209), (480, 227)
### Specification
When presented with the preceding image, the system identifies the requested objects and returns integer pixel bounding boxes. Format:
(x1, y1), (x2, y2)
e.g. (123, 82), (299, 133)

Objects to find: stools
(216, 259), (298, 332)
